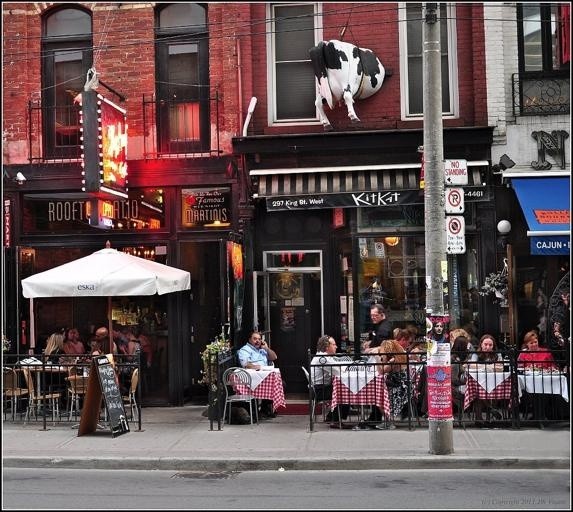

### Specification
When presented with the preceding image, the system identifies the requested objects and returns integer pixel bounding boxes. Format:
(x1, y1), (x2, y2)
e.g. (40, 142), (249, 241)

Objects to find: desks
(464, 371), (568, 430)
(230, 366), (281, 420)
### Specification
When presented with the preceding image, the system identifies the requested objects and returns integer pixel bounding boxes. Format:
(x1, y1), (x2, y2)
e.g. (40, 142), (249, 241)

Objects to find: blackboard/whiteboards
(94, 355), (128, 428)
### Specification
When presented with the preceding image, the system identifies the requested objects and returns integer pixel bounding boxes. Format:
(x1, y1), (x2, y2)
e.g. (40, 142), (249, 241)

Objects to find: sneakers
(324, 417), (396, 431)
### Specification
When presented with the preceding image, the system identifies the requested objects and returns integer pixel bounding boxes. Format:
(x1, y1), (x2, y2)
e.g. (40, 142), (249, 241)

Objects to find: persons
(432, 320), (448, 343)
(38, 326), (154, 422)
(362, 303), (393, 427)
(360, 325), (570, 426)
(238, 330), (279, 419)
(308, 334), (351, 429)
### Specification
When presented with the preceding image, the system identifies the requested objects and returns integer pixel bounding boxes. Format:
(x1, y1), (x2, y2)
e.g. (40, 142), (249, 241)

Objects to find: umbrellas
(20, 238), (193, 353)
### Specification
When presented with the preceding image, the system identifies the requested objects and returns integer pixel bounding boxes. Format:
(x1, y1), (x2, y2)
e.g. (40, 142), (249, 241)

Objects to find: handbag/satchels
(16, 355), (43, 369)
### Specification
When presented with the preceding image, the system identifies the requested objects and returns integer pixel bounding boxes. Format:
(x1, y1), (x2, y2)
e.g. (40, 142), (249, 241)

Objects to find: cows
(308, 39), (386, 132)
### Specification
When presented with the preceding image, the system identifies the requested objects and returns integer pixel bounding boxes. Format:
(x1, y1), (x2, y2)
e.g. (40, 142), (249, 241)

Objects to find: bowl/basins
(263, 366), (274, 371)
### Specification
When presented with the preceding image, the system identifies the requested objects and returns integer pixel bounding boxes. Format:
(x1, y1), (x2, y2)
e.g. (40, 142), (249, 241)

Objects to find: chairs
(221, 367), (259, 429)
(3, 367), (139, 428)
(301, 362), (425, 432)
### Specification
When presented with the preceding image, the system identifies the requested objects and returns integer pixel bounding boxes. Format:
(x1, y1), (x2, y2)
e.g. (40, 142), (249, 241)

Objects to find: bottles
(504, 356), (510, 372)
